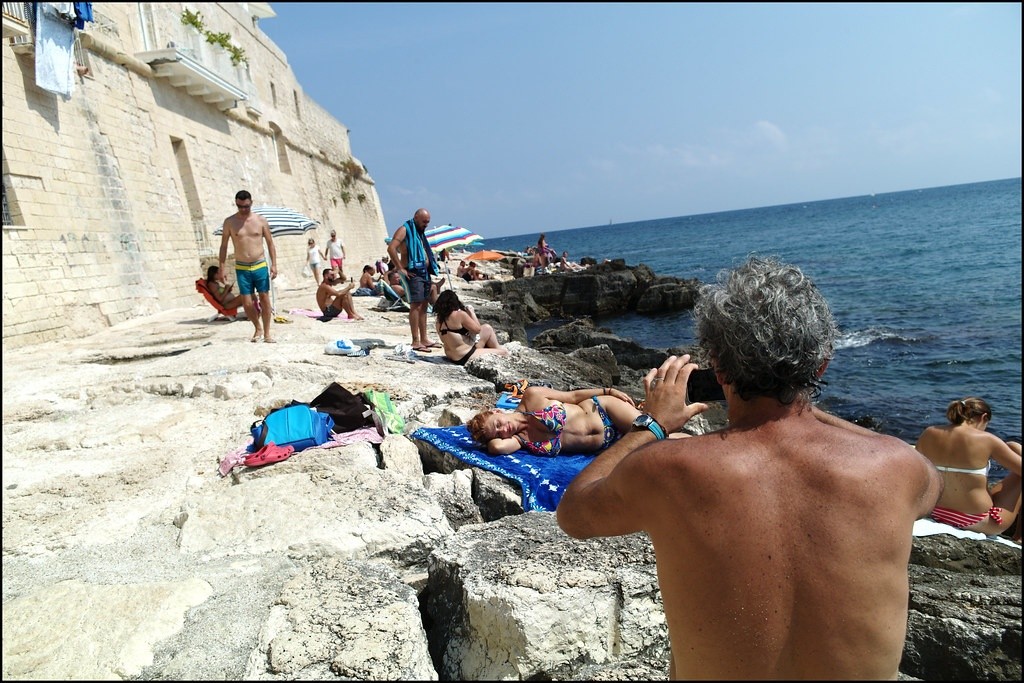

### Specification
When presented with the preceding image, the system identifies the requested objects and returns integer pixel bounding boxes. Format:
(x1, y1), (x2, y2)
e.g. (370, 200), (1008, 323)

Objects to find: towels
(911, 516), (1023, 549)
(42, 2), (94, 30)
(403, 219), (440, 277)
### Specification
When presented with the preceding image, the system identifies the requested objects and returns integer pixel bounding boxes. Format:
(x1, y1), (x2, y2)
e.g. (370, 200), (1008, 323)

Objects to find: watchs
(631, 414), (667, 443)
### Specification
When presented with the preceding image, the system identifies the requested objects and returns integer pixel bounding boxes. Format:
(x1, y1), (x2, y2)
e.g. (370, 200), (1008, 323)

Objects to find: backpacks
(249, 401), (335, 452)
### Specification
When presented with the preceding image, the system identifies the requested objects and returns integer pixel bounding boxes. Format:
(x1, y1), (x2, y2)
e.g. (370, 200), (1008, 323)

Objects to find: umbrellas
(465, 250), (505, 275)
(213, 204), (321, 317)
(423, 225), (483, 290)
(467, 241), (484, 253)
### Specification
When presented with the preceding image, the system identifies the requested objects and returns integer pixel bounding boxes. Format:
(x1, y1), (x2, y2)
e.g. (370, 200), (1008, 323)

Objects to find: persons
(556, 261), (945, 681)
(205, 188), (278, 343)
(526, 233), (587, 274)
(914, 397), (1022, 538)
(467, 386), (694, 457)
(305, 209), (512, 366)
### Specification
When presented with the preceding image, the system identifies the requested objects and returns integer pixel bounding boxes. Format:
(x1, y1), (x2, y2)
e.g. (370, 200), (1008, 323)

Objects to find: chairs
(378, 279), (432, 312)
(197, 279), (250, 324)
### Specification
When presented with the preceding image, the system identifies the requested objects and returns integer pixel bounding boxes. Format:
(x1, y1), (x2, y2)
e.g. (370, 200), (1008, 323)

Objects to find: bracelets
(603, 387), (612, 395)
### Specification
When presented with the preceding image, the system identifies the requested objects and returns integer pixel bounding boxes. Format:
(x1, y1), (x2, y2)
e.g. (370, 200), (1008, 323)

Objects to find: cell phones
(350, 277), (353, 283)
(686, 367), (726, 403)
(230, 283), (234, 289)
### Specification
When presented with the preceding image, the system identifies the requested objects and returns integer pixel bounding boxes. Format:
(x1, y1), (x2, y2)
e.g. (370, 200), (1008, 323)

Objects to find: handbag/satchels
(312, 382), (388, 439)
(301, 264), (312, 278)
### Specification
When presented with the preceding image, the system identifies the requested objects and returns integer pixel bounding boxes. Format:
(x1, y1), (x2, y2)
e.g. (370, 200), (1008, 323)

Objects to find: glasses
(237, 204), (250, 207)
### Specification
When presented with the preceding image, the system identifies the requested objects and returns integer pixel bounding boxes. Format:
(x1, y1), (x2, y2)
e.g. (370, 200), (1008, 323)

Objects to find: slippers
(426, 343), (442, 348)
(412, 345), (432, 352)
(244, 442), (293, 465)
